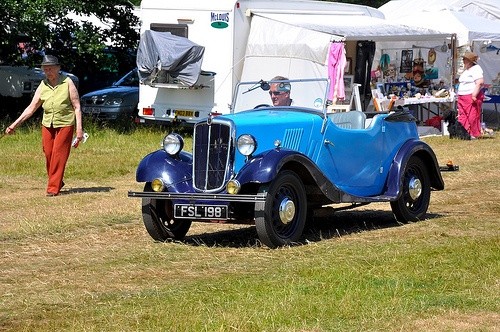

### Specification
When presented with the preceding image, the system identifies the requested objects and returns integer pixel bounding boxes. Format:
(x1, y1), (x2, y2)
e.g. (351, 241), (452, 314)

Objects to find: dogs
(443, 109), (471, 140)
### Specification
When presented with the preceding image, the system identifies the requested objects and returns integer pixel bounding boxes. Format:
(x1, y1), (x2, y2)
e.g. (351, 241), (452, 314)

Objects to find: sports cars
(127, 74), (460, 250)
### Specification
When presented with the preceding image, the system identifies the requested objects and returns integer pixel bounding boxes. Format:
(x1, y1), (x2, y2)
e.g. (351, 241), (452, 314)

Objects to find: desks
(374, 95), (500, 126)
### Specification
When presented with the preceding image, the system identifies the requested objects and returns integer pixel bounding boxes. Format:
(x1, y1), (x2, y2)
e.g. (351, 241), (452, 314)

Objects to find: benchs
(327, 110), (365, 130)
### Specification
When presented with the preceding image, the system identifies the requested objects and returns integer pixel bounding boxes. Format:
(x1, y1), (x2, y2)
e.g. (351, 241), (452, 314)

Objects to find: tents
(239, 0), (500, 138)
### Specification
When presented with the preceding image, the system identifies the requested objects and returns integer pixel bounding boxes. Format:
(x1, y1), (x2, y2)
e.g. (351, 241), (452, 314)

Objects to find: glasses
(269, 91), (283, 96)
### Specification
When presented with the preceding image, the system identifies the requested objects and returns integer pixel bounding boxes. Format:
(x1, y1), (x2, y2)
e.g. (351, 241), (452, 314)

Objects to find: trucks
(1, 42), (82, 119)
(138, 0), (470, 137)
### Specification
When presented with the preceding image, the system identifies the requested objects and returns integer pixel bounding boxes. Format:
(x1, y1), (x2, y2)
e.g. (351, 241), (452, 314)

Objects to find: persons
(269, 76), (295, 106)
(457, 51), (484, 138)
(4, 55), (83, 196)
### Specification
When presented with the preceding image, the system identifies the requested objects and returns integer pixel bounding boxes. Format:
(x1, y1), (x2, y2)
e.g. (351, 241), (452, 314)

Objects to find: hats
(40, 54), (60, 65)
(461, 49), (479, 64)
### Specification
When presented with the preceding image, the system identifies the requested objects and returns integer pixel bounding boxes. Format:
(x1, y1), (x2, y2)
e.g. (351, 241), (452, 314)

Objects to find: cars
(79, 65), (142, 131)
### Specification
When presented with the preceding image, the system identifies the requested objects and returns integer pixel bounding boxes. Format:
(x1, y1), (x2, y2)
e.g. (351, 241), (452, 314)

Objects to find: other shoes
(61, 183), (65, 188)
(46, 193), (57, 197)
